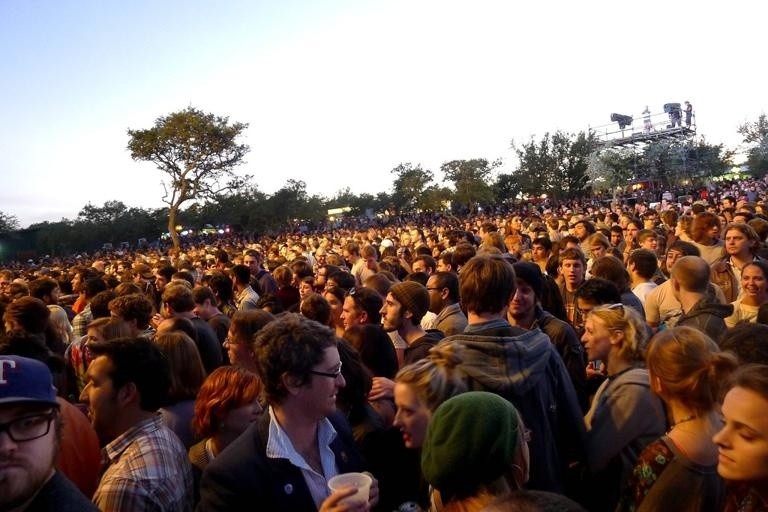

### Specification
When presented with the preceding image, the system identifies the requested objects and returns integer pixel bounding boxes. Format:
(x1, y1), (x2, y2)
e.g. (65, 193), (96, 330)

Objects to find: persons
(1, 169), (766, 511)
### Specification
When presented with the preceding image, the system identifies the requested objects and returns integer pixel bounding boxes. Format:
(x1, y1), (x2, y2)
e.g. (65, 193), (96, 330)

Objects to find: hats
(0, 355), (61, 408)
(421, 392), (519, 493)
(387, 281), (430, 323)
(132, 264), (154, 278)
(502, 252), (543, 299)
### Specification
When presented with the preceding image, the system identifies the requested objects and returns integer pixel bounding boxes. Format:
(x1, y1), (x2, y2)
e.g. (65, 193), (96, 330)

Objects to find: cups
(327, 471), (372, 505)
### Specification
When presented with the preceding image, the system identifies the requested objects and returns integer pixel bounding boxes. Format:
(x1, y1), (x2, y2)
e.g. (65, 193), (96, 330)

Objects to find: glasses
(348, 286), (364, 303)
(608, 302), (624, 315)
(309, 361), (342, 378)
(575, 308), (588, 315)
(0, 404), (56, 443)
(225, 336), (248, 344)
(520, 428), (533, 443)
